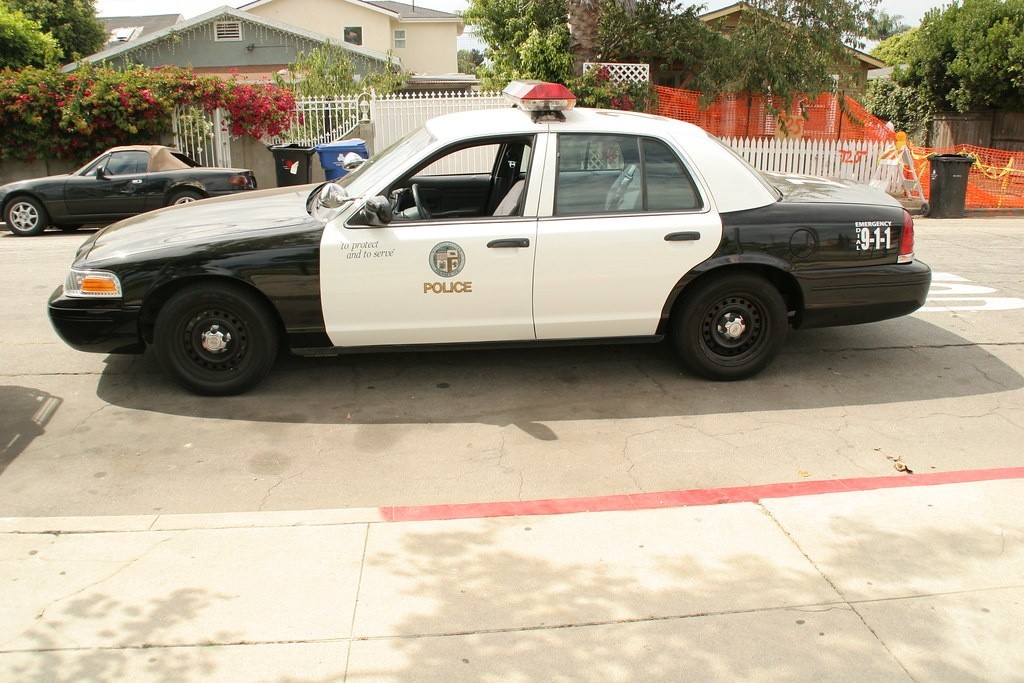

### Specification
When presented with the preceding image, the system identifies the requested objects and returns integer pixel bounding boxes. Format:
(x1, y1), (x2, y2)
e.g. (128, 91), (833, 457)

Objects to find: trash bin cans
(314, 138), (370, 181)
(266, 143), (317, 187)
(920, 154), (976, 218)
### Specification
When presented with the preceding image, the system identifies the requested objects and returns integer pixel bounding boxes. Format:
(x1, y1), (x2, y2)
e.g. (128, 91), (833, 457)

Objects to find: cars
(45, 81), (932, 396)
(0, 145), (260, 236)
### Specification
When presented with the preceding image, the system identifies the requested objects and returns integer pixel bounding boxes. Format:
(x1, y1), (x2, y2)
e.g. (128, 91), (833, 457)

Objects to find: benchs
(605, 163), (694, 210)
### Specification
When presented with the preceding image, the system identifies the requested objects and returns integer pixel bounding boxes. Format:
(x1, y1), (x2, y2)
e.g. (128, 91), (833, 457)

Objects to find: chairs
(431, 142), (525, 220)
